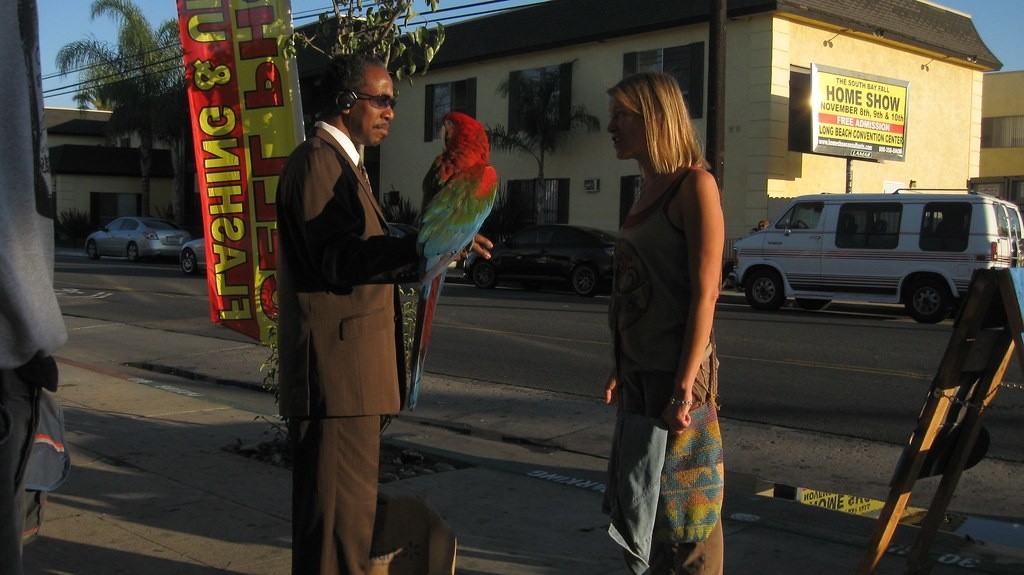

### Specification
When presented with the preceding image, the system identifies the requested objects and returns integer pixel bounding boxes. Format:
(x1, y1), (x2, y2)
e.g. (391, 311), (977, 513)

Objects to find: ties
(357, 159), (371, 192)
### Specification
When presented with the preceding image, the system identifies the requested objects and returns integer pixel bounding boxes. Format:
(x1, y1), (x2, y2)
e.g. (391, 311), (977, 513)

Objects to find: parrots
(407, 112), (497, 411)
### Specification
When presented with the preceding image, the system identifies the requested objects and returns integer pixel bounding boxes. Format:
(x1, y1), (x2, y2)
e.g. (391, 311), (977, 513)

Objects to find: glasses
(354, 90), (397, 110)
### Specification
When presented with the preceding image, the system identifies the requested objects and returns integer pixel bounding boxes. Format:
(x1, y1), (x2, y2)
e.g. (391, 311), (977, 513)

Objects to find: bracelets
(671, 397), (693, 405)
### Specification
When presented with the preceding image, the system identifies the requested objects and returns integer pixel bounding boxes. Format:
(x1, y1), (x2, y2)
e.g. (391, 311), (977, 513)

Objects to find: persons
(275, 55), (496, 573)
(750, 219), (769, 234)
(605, 72), (726, 574)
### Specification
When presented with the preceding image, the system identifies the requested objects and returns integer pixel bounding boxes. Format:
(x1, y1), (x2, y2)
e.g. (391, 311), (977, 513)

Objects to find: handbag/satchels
(652, 400), (724, 544)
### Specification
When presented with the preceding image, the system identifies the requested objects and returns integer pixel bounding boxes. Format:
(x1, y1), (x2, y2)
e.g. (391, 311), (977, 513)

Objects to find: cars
(180, 236), (206, 276)
(462, 223), (617, 299)
(85, 216), (192, 265)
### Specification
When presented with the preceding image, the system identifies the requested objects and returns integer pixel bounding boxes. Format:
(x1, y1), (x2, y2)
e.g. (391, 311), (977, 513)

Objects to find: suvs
(731, 184), (1024, 324)
